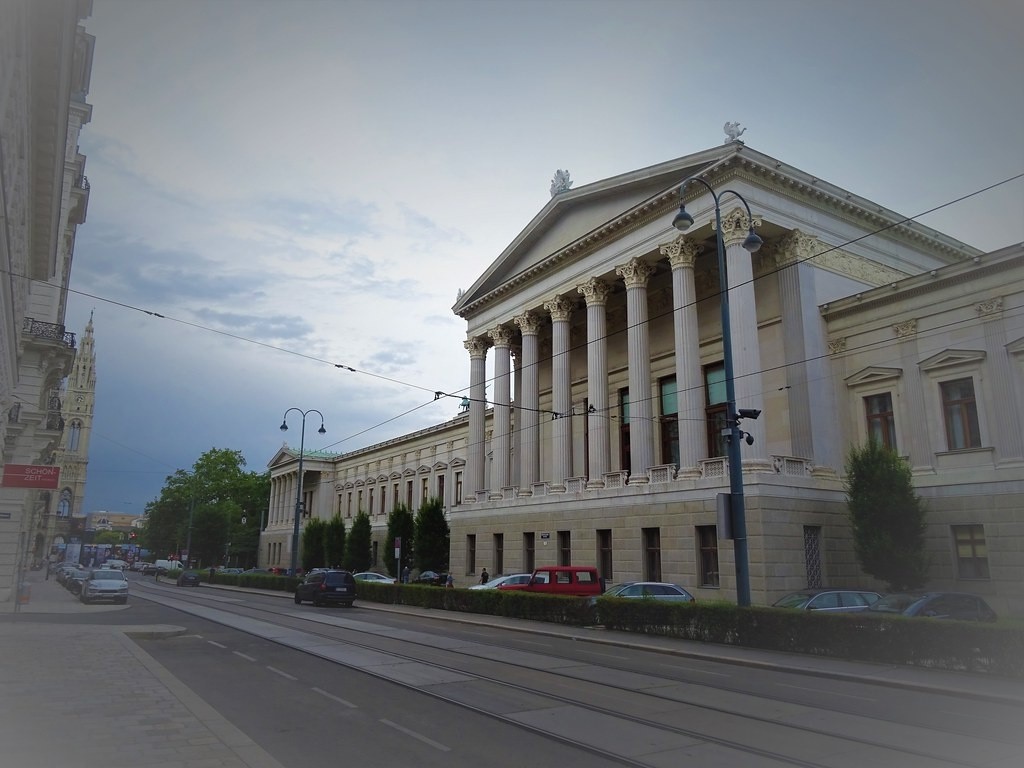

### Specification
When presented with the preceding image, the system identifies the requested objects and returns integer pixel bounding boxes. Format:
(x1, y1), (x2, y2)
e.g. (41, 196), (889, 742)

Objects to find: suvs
(295, 570), (358, 608)
(497, 566), (606, 596)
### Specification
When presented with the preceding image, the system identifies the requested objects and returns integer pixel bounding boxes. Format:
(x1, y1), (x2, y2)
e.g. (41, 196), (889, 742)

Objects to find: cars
(221, 569), (242, 574)
(240, 569), (270, 574)
(772, 588), (888, 612)
(177, 573), (200, 587)
(468, 574), (549, 590)
(353, 572), (398, 584)
(49, 562), (90, 591)
(861, 592), (999, 622)
(588, 582), (695, 625)
(81, 570), (129, 605)
(412, 570), (448, 586)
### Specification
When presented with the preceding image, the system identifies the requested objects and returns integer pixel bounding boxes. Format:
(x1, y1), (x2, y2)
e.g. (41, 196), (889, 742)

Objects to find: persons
(82, 577), (90, 604)
(154, 568), (161, 586)
(446, 571), (455, 588)
(479, 567), (489, 585)
(208, 564), (216, 584)
(403, 566), (410, 584)
(344, 568), (359, 608)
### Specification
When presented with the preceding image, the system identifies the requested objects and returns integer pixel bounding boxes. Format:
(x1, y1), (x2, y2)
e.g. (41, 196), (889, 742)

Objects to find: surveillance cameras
(745, 436), (754, 445)
(739, 408), (762, 419)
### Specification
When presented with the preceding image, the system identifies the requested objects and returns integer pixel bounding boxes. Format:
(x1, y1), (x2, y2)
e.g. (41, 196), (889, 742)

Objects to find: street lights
(672, 177), (765, 608)
(280, 408), (327, 575)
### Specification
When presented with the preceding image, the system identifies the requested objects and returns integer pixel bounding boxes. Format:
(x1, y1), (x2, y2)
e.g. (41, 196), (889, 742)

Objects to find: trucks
(155, 560), (184, 570)
(106, 560), (130, 570)
